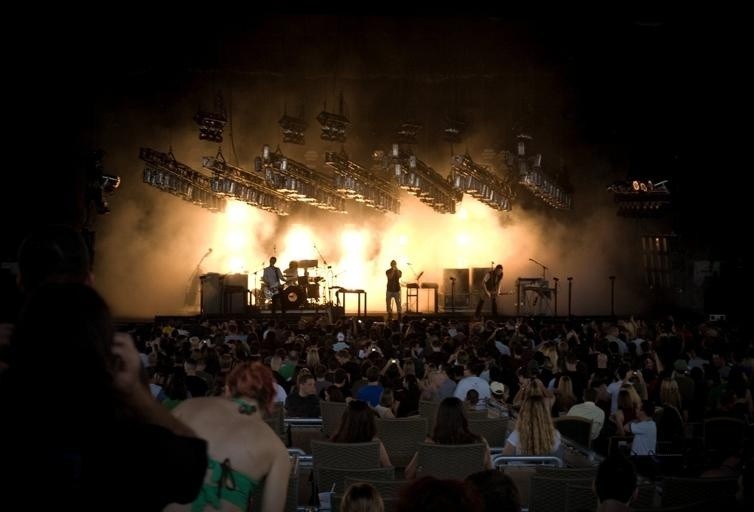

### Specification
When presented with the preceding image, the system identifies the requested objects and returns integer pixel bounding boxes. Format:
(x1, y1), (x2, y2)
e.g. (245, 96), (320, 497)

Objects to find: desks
(406, 283), (419, 313)
(336, 291), (367, 314)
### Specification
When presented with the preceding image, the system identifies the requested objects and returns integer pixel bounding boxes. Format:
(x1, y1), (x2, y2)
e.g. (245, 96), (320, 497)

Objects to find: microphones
(553, 278), (559, 281)
(274, 244), (276, 252)
(275, 269), (278, 272)
(568, 277), (572, 280)
(449, 277), (456, 280)
(204, 248), (212, 257)
(491, 261), (494, 264)
(609, 276), (616, 280)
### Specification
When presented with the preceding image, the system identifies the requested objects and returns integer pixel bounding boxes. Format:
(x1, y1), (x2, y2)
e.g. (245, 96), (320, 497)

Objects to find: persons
(476, 265), (504, 315)
(134, 314), (753, 512)
(3, 282), (207, 511)
(262, 257), (299, 314)
(386, 260), (402, 320)
(11, 234), (95, 287)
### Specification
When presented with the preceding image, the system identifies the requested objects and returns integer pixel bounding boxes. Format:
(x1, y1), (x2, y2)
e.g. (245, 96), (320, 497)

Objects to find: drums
(282, 286), (303, 310)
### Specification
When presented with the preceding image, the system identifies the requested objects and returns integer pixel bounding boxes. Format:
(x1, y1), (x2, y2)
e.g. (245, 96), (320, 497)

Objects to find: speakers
(441, 268), (470, 309)
(202, 273), (247, 313)
(471, 267), (493, 308)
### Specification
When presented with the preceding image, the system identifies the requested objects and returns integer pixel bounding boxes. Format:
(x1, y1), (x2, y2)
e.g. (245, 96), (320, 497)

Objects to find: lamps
(91, 172), (122, 215)
(631, 176), (654, 193)
(441, 112), (466, 135)
(398, 116), (421, 140)
(315, 112), (350, 140)
(195, 112), (226, 141)
(278, 113), (307, 144)
(138, 142), (574, 214)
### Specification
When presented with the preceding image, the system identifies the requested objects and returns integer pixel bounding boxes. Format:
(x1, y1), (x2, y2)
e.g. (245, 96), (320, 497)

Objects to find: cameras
(392, 360), (397, 363)
(202, 341), (207, 343)
(372, 347), (376, 351)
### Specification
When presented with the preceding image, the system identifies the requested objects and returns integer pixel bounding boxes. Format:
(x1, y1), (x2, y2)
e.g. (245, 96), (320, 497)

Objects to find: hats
(490, 381), (505, 395)
(337, 332), (345, 342)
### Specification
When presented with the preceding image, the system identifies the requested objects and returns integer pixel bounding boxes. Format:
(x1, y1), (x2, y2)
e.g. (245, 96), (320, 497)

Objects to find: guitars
(479, 290), (514, 301)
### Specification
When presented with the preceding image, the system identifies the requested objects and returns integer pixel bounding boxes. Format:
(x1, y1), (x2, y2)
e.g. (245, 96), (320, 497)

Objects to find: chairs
(119, 314), (750, 512)
